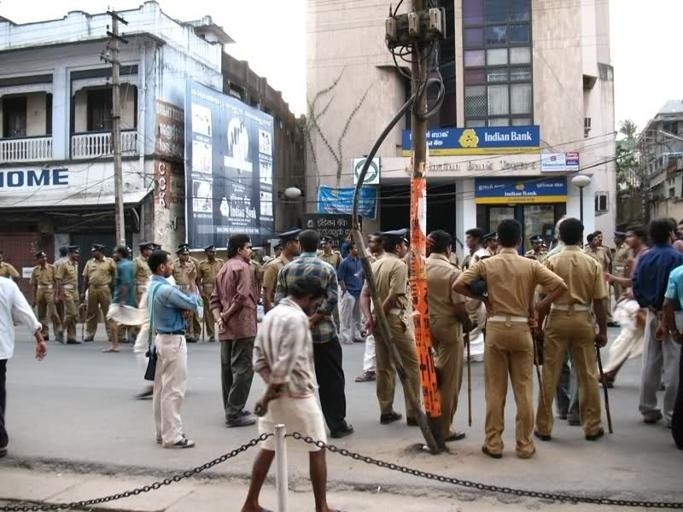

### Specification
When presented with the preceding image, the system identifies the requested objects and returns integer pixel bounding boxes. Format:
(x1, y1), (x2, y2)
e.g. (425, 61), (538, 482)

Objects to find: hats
(381, 228), (409, 244)
(274, 243), (283, 250)
(615, 232), (626, 239)
(33, 243), (216, 258)
(483, 232), (498, 240)
(529, 234), (544, 243)
(320, 236), (332, 244)
(277, 228), (302, 240)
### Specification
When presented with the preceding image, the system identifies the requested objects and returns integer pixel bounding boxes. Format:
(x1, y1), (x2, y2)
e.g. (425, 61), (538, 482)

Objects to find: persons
(262, 230), (354, 438)
(337, 213), (683, 460)
(0, 274), (50, 458)
(135, 250), (199, 447)
(32, 233), (342, 351)
(208, 234), (256, 426)
(0, 252), (22, 285)
(235, 273), (340, 511)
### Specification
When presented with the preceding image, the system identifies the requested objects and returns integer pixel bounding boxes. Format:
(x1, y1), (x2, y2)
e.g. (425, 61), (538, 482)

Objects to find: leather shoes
(43, 335), (215, 352)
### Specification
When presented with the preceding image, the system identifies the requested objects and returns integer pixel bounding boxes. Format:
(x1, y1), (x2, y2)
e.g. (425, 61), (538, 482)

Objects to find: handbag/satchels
(144, 347), (158, 380)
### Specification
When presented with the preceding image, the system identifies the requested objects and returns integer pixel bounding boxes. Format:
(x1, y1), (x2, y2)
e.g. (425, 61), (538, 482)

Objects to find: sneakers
(166, 437), (194, 447)
(135, 386), (154, 398)
(157, 433), (185, 444)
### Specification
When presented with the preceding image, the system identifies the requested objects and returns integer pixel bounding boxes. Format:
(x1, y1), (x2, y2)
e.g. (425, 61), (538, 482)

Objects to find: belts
(160, 330), (185, 334)
(551, 302), (590, 312)
(487, 315), (529, 323)
(388, 309), (402, 315)
